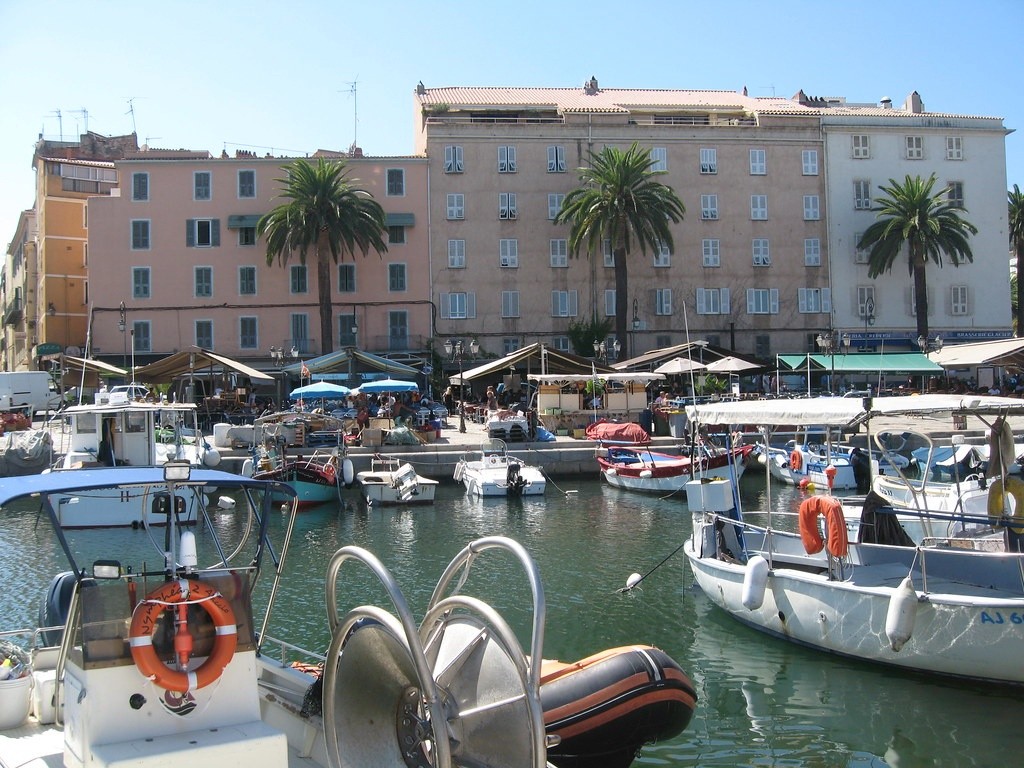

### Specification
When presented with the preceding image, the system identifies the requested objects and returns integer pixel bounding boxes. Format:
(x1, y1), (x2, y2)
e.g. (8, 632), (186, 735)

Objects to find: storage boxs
(686, 480), (735, 513)
(573, 429), (585, 438)
(557, 430), (568, 435)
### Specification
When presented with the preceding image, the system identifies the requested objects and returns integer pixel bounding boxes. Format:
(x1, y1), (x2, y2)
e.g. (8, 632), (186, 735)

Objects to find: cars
(110, 384), (157, 403)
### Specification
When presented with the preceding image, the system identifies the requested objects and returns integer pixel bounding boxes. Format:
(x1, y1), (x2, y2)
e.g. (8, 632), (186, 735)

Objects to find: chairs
(197, 401), (449, 432)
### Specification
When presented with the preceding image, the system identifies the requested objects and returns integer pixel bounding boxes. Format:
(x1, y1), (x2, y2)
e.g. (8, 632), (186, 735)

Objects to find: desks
(458, 401), (640, 431)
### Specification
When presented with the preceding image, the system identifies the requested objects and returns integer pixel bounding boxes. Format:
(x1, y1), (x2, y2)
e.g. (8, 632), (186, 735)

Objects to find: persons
(251, 385), (525, 425)
(752, 374), (785, 393)
(586, 395), (604, 410)
(652, 381), (684, 407)
(683, 419), (690, 445)
(907, 372), (1024, 395)
(795, 425), (805, 445)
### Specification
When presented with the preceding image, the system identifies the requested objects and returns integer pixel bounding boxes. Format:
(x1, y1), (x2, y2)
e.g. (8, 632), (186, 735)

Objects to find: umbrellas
(701, 355), (766, 393)
(290, 378), (352, 416)
(654, 356), (708, 374)
(359, 376), (418, 429)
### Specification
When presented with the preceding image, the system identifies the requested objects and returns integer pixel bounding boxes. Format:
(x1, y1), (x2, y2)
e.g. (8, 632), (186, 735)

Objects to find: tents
(43, 341), (734, 387)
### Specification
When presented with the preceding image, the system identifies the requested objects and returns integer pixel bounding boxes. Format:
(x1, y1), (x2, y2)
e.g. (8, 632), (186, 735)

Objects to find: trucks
(1, 369), (69, 419)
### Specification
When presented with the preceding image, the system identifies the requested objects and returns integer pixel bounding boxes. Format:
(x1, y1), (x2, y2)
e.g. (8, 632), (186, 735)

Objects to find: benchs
(88, 721), (290, 768)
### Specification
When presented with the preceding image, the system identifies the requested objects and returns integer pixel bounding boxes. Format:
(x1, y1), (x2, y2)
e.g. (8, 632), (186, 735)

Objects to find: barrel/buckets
(417, 424), (440, 442)
(952, 416), (967, 429)
(0, 674), (31, 730)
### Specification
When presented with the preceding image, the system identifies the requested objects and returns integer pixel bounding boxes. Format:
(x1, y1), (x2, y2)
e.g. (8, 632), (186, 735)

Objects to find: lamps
(48, 302), (56, 316)
(960, 399), (981, 409)
(93, 560), (121, 579)
(163, 462), (191, 482)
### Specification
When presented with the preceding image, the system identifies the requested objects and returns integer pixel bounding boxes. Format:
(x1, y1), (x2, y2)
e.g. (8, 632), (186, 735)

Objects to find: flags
(302, 361), (311, 378)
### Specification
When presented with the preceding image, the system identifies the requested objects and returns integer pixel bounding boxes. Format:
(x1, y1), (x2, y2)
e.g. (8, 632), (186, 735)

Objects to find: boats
(41, 299), (221, 529)
(583, 297), (1024, 550)
(355, 453), (440, 507)
(456, 437), (547, 498)
(681, 298), (1024, 689)
(524, 642), (698, 768)
(0, 464), (562, 768)
(240, 421), (354, 512)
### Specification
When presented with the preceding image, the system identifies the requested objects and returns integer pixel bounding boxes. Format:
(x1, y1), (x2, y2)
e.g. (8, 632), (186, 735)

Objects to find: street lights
(815, 332), (852, 397)
(917, 334), (943, 395)
(269, 345), (300, 411)
(443, 338), (480, 433)
(592, 339), (622, 366)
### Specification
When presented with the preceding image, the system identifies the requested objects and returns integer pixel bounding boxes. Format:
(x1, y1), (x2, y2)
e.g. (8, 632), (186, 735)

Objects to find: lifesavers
(987, 476), (1024, 534)
(799, 495), (848, 558)
(790, 451), (802, 470)
(129, 578), (237, 692)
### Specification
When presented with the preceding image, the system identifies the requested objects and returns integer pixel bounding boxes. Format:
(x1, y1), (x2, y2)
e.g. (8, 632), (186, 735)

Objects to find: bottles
(217, 495), (236, 509)
(0, 659), (23, 680)
